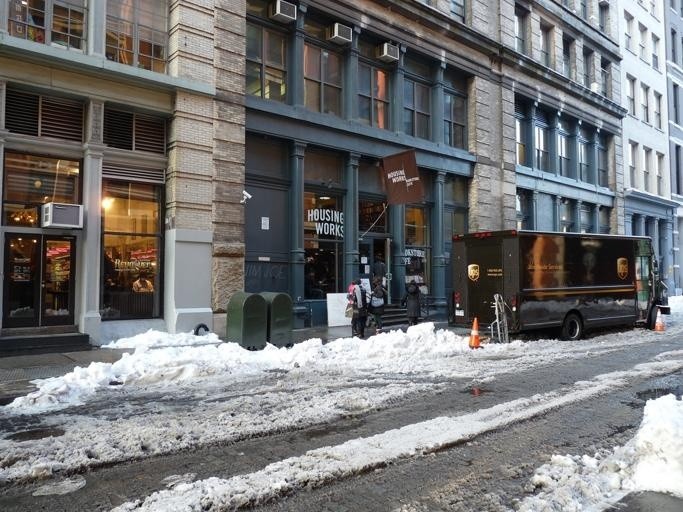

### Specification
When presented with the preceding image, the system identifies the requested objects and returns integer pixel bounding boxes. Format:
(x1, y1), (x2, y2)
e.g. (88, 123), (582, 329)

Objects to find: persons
(399, 279), (423, 325)
(131, 271), (152, 292)
(345, 251), (386, 339)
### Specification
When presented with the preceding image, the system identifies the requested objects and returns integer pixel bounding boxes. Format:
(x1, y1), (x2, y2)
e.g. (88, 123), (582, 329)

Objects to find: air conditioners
(39, 202), (83, 229)
(267, 0), (399, 64)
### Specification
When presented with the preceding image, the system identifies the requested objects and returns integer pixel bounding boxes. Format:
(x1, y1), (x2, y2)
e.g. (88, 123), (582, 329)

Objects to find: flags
(379, 147), (425, 207)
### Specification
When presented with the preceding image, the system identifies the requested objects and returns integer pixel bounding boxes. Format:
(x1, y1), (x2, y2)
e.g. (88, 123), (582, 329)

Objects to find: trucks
(448, 230), (671, 341)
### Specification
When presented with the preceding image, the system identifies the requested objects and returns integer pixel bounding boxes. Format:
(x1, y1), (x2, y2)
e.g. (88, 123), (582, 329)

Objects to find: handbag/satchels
(371, 296), (384, 308)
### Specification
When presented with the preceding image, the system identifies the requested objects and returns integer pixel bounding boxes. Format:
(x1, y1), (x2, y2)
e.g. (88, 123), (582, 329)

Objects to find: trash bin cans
(226, 292), (293, 351)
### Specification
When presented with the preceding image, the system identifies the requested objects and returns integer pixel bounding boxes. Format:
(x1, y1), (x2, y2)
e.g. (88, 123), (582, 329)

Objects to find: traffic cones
(466, 315), (484, 350)
(651, 304), (665, 334)
